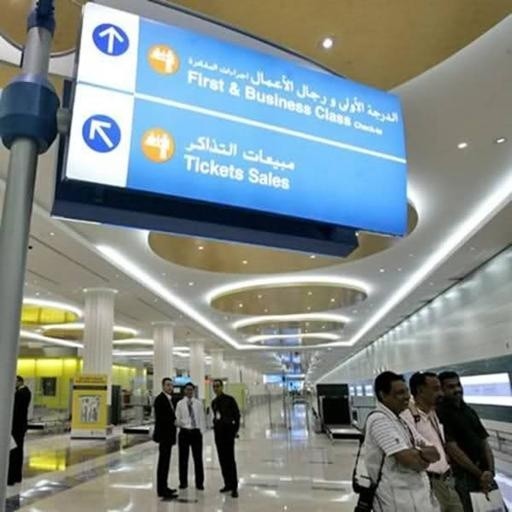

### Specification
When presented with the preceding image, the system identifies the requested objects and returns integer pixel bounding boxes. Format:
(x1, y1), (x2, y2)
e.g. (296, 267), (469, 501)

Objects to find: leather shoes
(160, 484), (204, 497)
(220, 486), (237, 497)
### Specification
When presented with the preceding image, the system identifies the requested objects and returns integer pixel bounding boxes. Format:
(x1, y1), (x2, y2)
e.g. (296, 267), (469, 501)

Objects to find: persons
(6, 375), (32, 486)
(174, 382), (205, 491)
(211, 379), (241, 498)
(152, 377), (179, 500)
(362, 370), (509, 511)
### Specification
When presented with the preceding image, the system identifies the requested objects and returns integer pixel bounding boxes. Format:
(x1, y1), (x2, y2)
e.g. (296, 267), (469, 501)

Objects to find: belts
(429, 469), (452, 481)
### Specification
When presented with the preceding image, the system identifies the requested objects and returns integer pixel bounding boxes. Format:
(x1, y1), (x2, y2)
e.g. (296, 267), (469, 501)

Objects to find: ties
(187, 401), (197, 427)
